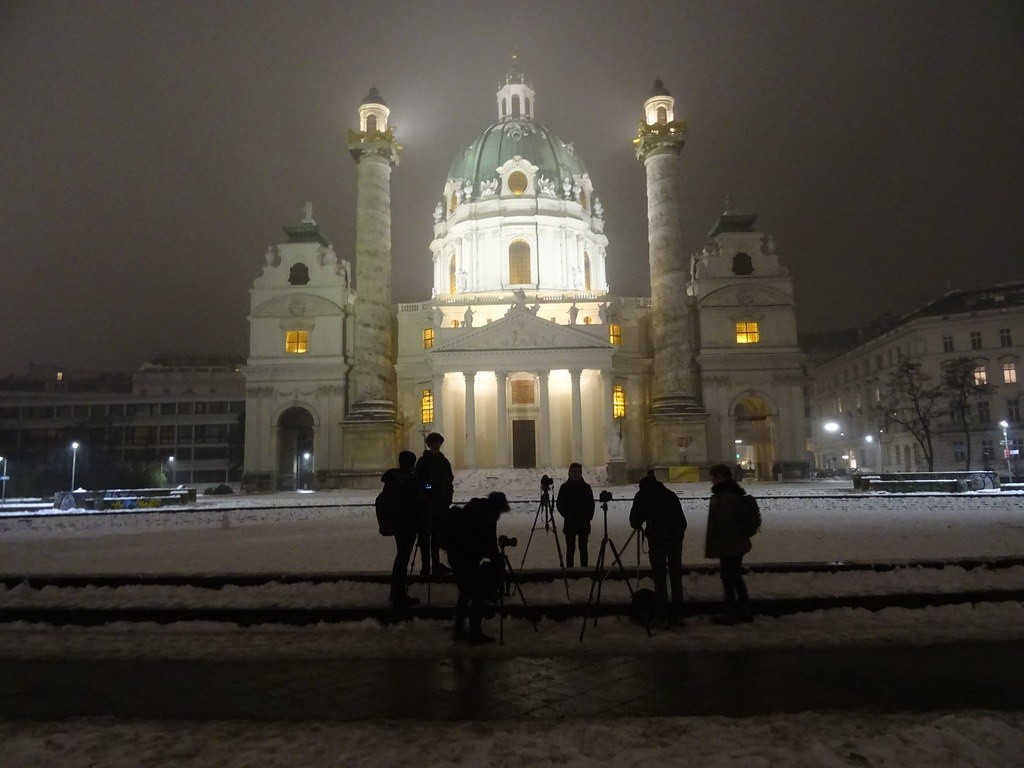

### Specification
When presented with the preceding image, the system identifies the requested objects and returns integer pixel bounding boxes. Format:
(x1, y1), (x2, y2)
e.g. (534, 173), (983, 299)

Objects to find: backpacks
(375, 491), (394, 536)
(739, 489), (761, 538)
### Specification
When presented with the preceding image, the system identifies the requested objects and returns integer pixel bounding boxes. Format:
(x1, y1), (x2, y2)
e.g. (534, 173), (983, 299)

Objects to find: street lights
(0, 456), (8, 503)
(70, 441), (79, 492)
(998, 420), (1012, 484)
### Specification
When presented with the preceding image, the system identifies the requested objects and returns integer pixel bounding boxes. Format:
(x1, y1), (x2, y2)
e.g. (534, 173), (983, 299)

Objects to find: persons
(705, 465), (754, 625)
(598, 301), (608, 323)
(464, 305), (477, 326)
(323, 242), (337, 264)
(541, 173), (555, 191)
(482, 178), (496, 191)
(413, 432), (454, 576)
(433, 180), (473, 219)
(556, 463), (595, 567)
(563, 178), (582, 202)
(381, 451), (427, 607)
(431, 305), (447, 328)
(565, 302), (583, 324)
(448, 491), (512, 644)
(593, 197), (604, 214)
(512, 286), (526, 307)
(629, 475), (687, 631)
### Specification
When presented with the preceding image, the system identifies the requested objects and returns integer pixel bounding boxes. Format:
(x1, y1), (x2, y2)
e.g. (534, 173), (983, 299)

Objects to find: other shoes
(450, 626), (467, 641)
(432, 563), (449, 573)
(391, 594), (420, 606)
(420, 565), (430, 576)
(468, 632), (496, 646)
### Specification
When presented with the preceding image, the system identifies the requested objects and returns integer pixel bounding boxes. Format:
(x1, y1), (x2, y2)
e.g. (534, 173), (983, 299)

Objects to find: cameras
(540, 475), (553, 486)
(498, 535), (517, 548)
(599, 491), (612, 502)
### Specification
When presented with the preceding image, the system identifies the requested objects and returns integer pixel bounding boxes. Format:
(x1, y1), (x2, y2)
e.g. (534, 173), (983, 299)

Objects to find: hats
(489, 492), (510, 512)
(639, 470), (657, 487)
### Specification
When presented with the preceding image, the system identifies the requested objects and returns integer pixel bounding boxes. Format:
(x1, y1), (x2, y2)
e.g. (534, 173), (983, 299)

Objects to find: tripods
(406, 486), (652, 647)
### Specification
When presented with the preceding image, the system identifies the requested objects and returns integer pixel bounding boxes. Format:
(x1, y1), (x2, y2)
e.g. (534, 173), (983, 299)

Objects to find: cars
(816, 468), (835, 478)
(849, 467), (875, 479)
